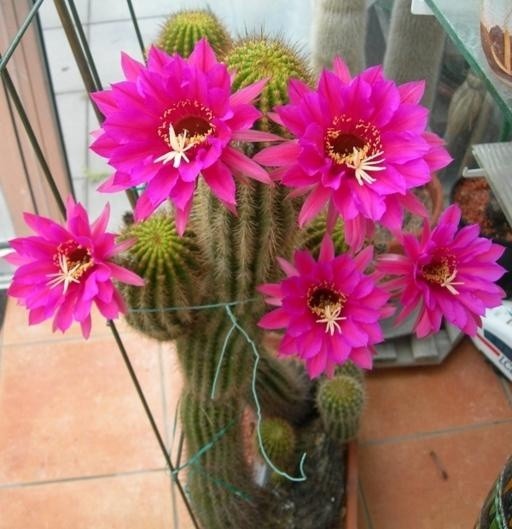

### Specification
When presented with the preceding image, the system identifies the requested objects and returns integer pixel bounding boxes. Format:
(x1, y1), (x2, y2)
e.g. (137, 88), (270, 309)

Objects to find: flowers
(3, 8), (509, 525)
